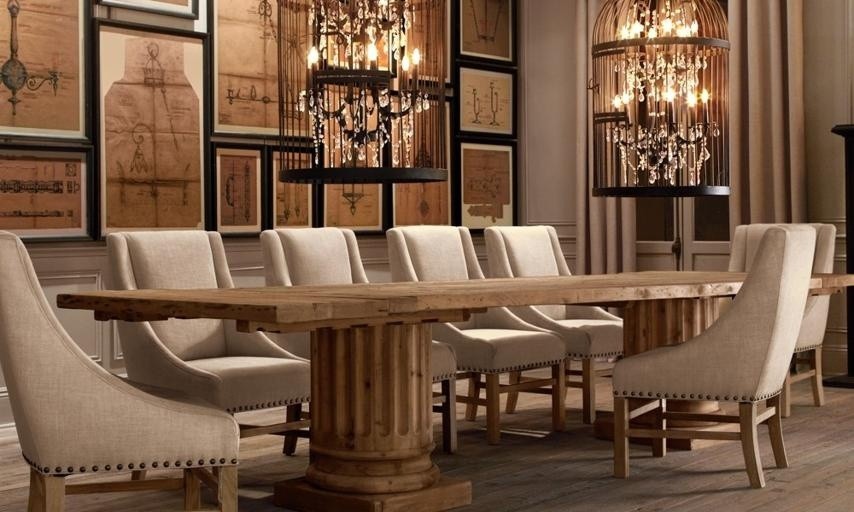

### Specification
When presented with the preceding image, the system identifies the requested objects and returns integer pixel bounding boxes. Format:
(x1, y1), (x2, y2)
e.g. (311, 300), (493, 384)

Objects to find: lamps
(274, 0), (449, 185)
(590, 0), (731, 199)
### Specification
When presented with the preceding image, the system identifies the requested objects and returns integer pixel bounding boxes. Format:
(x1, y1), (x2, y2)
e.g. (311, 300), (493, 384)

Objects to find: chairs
(610, 224), (818, 488)
(484, 225), (625, 425)
(744, 221), (836, 418)
(1, 229), (241, 511)
(259, 227), (458, 456)
(106, 229), (311, 481)
(728, 224), (747, 275)
(384, 225), (566, 445)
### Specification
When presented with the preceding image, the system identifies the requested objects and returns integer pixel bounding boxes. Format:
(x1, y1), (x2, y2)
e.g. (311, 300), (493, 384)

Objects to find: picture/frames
(451, 55), (520, 141)
(266, 144), (319, 230)
(450, 0), (520, 71)
(95, 0), (199, 19)
(320, 183), (380, 229)
(0, 143), (89, 244)
(0, 0), (91, 145)
(393, 181), (449, 225)
(92, 16), (210, 243)
(449, 140), (518, 234)
(206, 0), (317, 143)
(210, 140), (265, 238)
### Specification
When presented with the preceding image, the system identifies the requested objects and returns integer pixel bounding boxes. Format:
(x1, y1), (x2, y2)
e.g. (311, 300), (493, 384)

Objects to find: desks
(54, 268), (853, 510)
